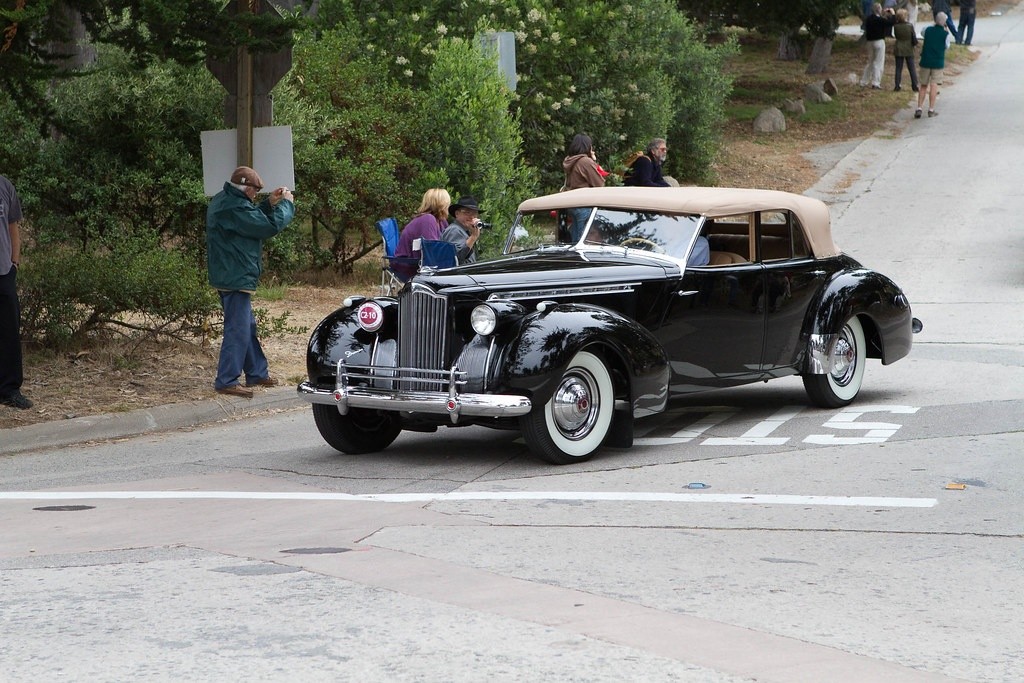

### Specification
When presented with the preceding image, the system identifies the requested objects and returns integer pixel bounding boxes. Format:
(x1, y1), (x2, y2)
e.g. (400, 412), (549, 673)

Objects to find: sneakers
(246, 376), (278, 386)
(214, 383), (254, 397)
(0, 390), (33, 409)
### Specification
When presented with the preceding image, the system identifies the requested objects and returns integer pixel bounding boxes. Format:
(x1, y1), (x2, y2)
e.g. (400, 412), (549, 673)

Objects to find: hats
(231, 166), (263, 188)
(448, 195), (487, 217)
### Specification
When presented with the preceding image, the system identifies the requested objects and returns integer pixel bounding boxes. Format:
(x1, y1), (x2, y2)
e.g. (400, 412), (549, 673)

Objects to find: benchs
(707, 234), (786, 262)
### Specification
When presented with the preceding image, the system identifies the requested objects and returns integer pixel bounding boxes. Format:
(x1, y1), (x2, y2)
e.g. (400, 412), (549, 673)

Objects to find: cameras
(476, 221), (492, 230)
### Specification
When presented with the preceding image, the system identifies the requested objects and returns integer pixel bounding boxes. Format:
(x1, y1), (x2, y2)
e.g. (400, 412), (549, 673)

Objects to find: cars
(298, 185), (922, 466)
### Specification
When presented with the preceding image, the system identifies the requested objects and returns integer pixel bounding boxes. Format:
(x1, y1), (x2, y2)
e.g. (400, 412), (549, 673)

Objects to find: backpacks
(623, 151), (651, 178)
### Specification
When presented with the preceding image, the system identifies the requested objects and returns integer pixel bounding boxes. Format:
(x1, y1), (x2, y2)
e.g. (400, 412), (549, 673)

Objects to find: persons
(391, 188), (452, 283)
(0, 176), (33, 408)
(862, 1), (975, 46)
(576, 217), (711, 268)
(617, 138), (671, 187)
(894, 8), (919, 92)
(437, 195), (487, 268)
(205, 166), (294, 399)
(859, 3), (896, 91)
(561, 134), (608, 244)
(914, 11), (950, 118)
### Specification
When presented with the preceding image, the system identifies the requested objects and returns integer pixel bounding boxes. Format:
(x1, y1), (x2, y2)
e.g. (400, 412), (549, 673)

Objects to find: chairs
(708, 252), (744, 267)
(376, 216), (419, 298)
(420, 236), (458, 268)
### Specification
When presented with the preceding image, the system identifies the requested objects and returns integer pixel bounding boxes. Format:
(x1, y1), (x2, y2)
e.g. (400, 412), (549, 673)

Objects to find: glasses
(456, 209), (479, 216)
(249, 186), (261, 192)
(659, 147), (667, 151)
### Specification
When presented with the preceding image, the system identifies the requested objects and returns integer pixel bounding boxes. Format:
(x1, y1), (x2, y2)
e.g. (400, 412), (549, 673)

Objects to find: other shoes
(915, 110), (922, 117)
(928, 110), (937, 117)
(913, 85), (920, 91)
(894, 86), (902, 92)
(872, 85), (883, 89)
